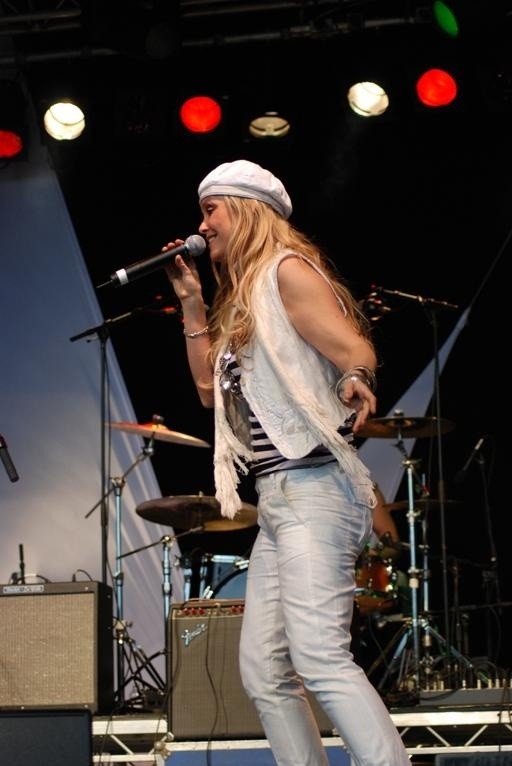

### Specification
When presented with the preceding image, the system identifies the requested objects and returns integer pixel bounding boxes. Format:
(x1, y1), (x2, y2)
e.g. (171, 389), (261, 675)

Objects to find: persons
(162, 158), (412, 766)
(371, 482), (401, 559)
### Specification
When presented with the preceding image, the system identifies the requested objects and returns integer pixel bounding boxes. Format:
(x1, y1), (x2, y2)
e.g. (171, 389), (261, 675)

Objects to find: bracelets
(334, 364), (379, 412)
(183, 326), (209, 338)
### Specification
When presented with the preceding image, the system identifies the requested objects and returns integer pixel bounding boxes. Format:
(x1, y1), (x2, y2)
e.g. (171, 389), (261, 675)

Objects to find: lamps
(1, 1), (512, 156)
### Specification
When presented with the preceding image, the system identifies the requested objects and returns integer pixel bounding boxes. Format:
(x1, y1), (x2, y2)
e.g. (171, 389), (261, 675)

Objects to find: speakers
(0, 581), (112, 715)
(0, 709), (92, 766)
(169, 598), (342, 742)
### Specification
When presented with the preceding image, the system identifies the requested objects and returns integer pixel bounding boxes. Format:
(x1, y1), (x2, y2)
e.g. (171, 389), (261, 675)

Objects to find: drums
(355, 555), (396, 615)
(204, 559), (248, 599)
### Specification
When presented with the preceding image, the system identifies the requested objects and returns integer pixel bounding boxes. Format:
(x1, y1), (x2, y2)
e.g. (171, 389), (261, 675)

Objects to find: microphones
(365, 281), (430, 321)
(110, 234), (207, 289)
(69, 305), (146, 343)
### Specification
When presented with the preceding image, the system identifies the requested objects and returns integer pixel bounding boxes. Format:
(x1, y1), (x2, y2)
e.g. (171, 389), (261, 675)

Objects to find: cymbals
(104, 421), (208, 446)
(384, 498), (453, 512)
(352, 415), (448, 438)
(136, 495), (259, 534)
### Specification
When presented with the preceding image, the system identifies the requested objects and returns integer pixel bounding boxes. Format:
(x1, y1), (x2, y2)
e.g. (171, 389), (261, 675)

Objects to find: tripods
(365, 458), (494, 692)
(111, 475), (172, 713)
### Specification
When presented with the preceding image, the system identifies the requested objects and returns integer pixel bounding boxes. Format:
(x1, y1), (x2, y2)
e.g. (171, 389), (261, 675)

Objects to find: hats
(197, 159), (293, 220)
